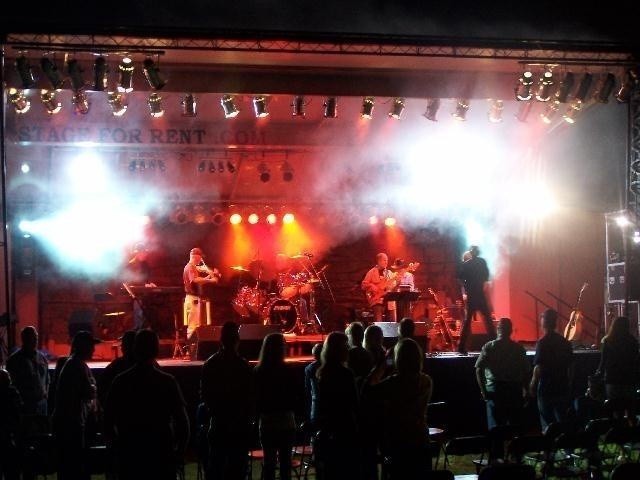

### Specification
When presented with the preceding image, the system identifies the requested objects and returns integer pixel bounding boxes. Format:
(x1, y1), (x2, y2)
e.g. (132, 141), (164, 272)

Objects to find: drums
(292, 297), (307, 320)
(247, 288), (269, 318)
(263, 299), (299, 333)
(232, 286), (252, 318)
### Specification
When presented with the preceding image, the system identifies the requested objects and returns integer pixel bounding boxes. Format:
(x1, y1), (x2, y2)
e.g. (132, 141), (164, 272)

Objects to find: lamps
(1, 40), (166, 94)
(122, 143), (177, 176)
(515, 57), (632, 105)
(195, 144), (237, 176)
(161, 200), (400, 228)
(7, 87), (630, 126)
(254, 148), (300, 185)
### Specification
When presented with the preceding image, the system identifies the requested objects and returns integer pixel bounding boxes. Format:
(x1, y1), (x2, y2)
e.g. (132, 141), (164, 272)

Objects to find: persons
(2, 322), (302, 479)
(124, 242), (159, 331)
(476, 310), (639, 453)
(361, 252), (397, 323)
(389, 257), (422, 322)
(460, 250), (497, 321)
(307, 319), (433, 480)
(183, 247), (219, 355)
(458, 245), (499, 356)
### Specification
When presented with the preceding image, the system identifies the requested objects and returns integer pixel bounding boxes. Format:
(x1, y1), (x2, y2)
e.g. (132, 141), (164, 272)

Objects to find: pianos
(124, 285), (184, 300)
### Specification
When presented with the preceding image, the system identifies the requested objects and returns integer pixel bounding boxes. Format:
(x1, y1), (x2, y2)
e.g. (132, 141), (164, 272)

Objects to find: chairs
(0, 401), (640, 480)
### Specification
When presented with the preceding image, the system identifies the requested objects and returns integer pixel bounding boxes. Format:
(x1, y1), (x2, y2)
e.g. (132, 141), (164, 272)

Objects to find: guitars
(365, 263), (420, 308)
(564, 282), (590, 342)
(192, 268), (222, 296)
(428, 287), (458, 350)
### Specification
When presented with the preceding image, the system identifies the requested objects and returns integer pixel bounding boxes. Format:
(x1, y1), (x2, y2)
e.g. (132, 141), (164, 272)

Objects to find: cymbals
(288, 255), (308, 259)
(307, 278), (323, 283)
(229, 267), (249, 271)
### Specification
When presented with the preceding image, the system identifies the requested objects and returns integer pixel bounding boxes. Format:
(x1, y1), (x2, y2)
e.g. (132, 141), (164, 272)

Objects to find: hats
(391, 258), (405, 269)
(190, 248), (206, 258)
(71, 331), (101, 345)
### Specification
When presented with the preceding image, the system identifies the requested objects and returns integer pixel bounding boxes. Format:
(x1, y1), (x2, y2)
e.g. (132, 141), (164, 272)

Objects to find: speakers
(372, 322), (426, 357)
(187, 326), (223, 360)
(236, 325), (282, 360)
(12, 237), (35, 281)
(604, 303), (621, 337)
(621, 303), (640, 339)
(605, 209), (640, 326)
(461, 319), (501, 351)
(68, 308), (98, 338)
